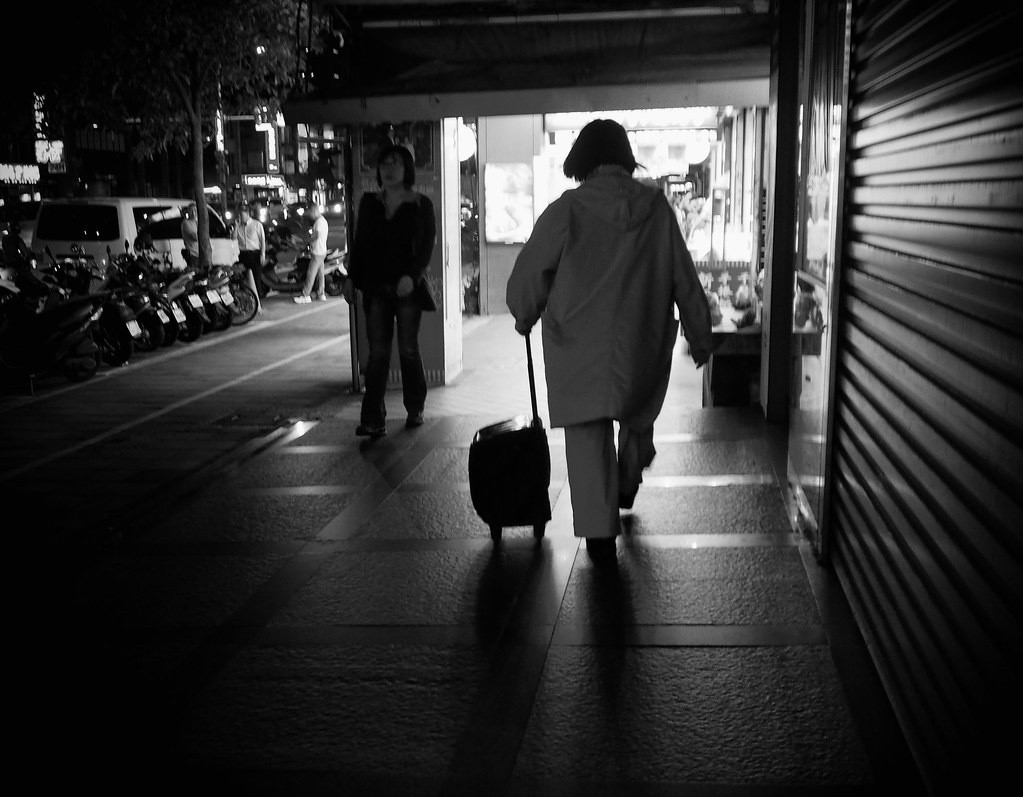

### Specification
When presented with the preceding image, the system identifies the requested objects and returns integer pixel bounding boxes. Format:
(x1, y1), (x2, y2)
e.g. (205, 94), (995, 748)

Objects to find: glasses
(378, 161), (404, 168)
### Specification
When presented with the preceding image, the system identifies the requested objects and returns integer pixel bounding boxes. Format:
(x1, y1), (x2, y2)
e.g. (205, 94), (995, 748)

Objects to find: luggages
(468, 332), (550, 546)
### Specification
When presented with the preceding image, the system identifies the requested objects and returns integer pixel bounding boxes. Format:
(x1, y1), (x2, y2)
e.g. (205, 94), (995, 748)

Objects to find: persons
(506, 120), (714, 569)
(230, 204), (266, 299)
(180, 203), (200, 270)
(294, 203), (329, 304)
(349, 146), (436, 437)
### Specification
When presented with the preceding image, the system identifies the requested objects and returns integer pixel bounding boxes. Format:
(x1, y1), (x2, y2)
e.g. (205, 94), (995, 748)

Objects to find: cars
(206, 196), (346, 228)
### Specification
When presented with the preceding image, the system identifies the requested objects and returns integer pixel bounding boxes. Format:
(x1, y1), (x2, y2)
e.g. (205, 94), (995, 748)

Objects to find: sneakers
(293, 293), (327, 304)
(355, 405), (426, 437)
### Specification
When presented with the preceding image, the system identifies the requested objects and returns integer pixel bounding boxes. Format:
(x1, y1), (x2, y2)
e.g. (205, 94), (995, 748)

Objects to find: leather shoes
(586, 500), (638, 565)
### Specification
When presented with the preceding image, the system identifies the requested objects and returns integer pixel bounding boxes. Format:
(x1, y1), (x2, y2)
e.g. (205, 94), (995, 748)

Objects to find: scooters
(256, 230), (348, 296)
(0, 236), (260, 397)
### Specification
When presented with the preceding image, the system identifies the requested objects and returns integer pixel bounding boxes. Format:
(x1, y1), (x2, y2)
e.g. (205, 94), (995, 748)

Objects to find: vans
(32, 194), (241, 276)
